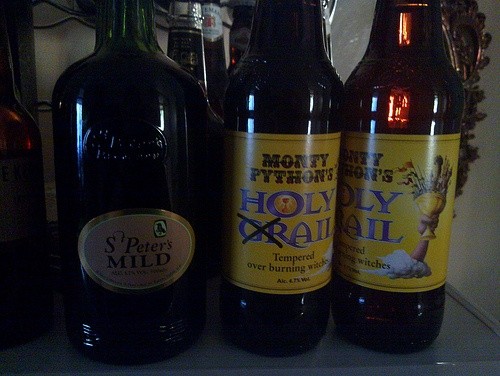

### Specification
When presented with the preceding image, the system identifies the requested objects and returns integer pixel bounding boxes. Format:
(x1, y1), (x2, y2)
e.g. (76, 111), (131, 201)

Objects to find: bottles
(52, 0), (206, 368)
(0, 14), (54, 351)
(220, 0), (344, 359)
(332, 0), (466, 355)
(165, 0), (256, 278)
